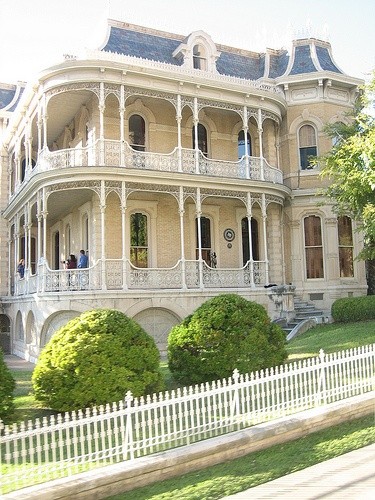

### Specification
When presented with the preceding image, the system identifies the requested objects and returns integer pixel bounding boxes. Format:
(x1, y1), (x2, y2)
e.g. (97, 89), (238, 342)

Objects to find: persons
(16, 259), (24, 279)
(210, 250), (217, 268)
(66, 254), (76, 270)
(76, 250), (87, 268)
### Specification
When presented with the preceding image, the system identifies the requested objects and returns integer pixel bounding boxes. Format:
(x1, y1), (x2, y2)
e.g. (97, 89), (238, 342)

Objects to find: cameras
(63, 260), (68, 264)
